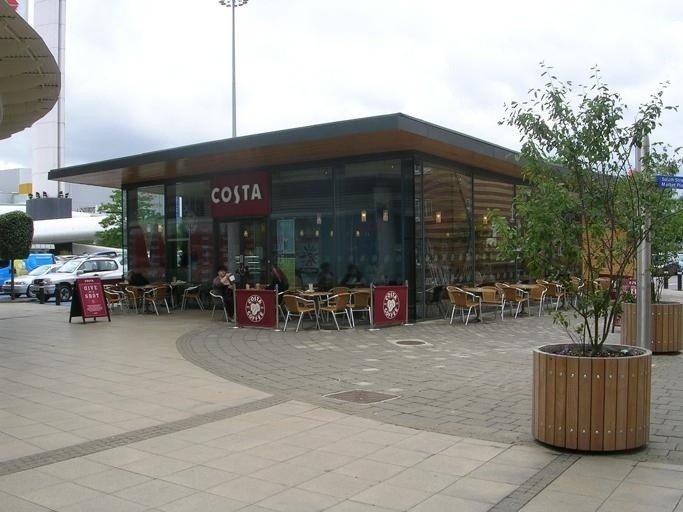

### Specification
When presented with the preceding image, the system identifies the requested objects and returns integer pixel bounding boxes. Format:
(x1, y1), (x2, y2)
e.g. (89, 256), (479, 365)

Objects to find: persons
(264, 264), (289, 305)
(212, 264), (235, 323)
(316, 261), (337, 285)
(124, 269), (153, 308)
(340, 264), (362, 285)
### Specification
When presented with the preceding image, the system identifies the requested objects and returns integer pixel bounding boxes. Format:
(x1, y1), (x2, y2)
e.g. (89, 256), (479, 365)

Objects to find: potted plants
(102, 278), (372, 332)
(482, 59), (683, 452)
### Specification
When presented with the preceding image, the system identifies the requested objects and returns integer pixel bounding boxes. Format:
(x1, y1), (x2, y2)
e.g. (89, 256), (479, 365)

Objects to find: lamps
(446, 277), (613, 325)
(434, 211), (442, 224)
(382, 209), (389, 223)
(423, 281), (495, 319)
(359, 209), (367, 222)
(316, 213), (322, 224)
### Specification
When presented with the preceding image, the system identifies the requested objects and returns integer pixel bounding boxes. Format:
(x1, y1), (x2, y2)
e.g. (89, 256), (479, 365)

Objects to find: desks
(218, 0), (249, 140)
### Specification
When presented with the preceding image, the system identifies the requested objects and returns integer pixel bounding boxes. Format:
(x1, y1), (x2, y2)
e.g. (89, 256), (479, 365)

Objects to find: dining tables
(102, 278), (372, 332)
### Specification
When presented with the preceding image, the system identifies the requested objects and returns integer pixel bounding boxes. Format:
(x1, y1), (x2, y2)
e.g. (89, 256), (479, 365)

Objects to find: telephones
(653, 175), (682, 189)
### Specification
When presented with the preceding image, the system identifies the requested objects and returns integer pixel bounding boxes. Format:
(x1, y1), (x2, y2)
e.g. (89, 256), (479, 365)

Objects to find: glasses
(382, 209), (389, 223)
(359, 209), (367, 222)
(316, 213), (322, 224)
(434, 211), (442, 224)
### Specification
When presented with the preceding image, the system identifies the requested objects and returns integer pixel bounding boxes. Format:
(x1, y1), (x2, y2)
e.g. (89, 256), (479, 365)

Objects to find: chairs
(423, 281), (495, 319)
(446, 277), (613, 325)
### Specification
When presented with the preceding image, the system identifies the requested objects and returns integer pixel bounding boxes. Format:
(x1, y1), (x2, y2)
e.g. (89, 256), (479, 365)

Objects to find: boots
(31, 256), (123, 303)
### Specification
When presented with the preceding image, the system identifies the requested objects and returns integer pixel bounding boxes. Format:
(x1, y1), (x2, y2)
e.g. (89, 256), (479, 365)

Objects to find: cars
(172, 277), (176, 283)
(255, 283), (261, 289)
(308, 283), (312, 291)
(245, 284), (249, 289)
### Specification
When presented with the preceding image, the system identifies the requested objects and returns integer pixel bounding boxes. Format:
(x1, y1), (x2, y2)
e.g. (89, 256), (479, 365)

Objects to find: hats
(649, 250), (683, 275)
(1, 264), (64, 298)
(55, 250), (182, 273)
